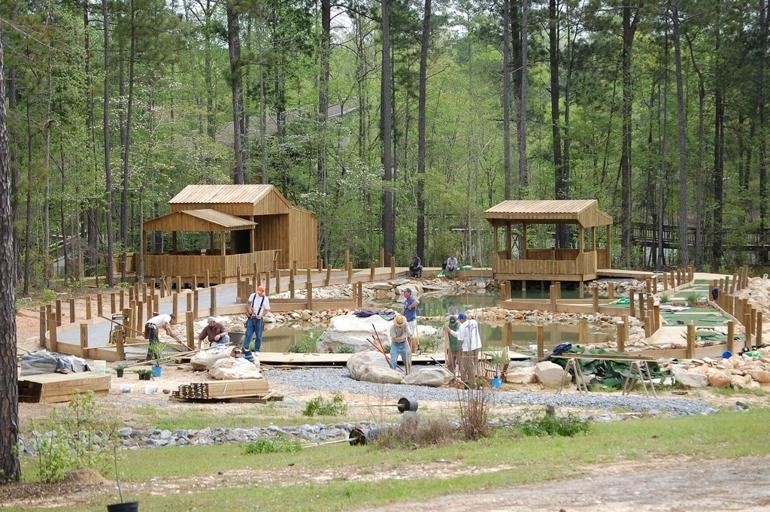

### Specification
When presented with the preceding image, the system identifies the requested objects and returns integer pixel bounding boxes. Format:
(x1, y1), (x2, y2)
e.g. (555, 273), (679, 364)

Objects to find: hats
(207, 318), (215, 323)
(450, 314), (465, 322)
(402, 288), (411, 292)
(257, 287), (264, 293)
(394, 315), (406, 326)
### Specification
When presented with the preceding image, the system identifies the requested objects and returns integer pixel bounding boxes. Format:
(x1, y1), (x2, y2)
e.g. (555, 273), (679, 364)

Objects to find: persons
(197, 316), (231, 350)
(401, 287), (419, 355)
(445, 315), (463, 375)
(240, 286), (271, 352)
(144, 313), (176, 361)
(445, 312), (482, 385)
(390, 315), (413, 375)
(409, 251), (424, 278)
(446, 255), (458, 273)
